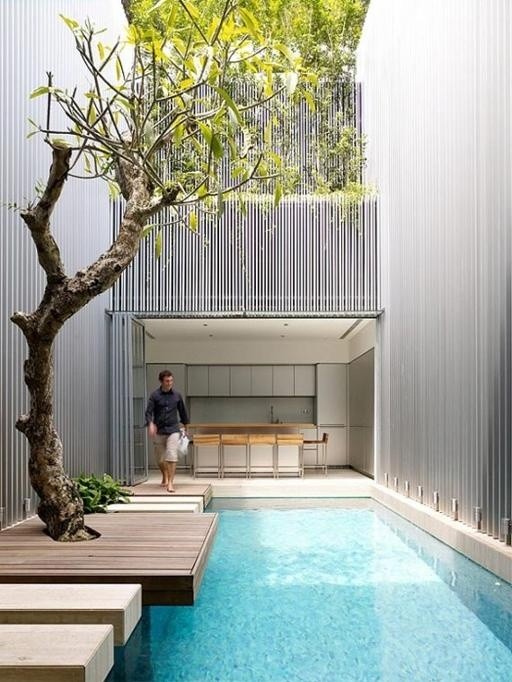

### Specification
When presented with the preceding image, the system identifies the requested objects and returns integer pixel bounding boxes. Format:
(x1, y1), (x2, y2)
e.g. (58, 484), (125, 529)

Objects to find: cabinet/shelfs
(146, 362), (349, 469)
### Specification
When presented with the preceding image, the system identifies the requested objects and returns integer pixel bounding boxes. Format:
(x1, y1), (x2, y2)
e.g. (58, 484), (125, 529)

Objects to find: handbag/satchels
(177, 435), (190, 454)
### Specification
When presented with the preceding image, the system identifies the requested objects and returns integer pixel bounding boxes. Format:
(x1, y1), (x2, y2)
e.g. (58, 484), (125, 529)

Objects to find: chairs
(193, 433), (304, 478)
(304, 432), (329, 475)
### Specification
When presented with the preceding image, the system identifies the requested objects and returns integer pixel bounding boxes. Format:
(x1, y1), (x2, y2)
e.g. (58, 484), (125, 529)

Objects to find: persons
(145, 370), (191, 492)
